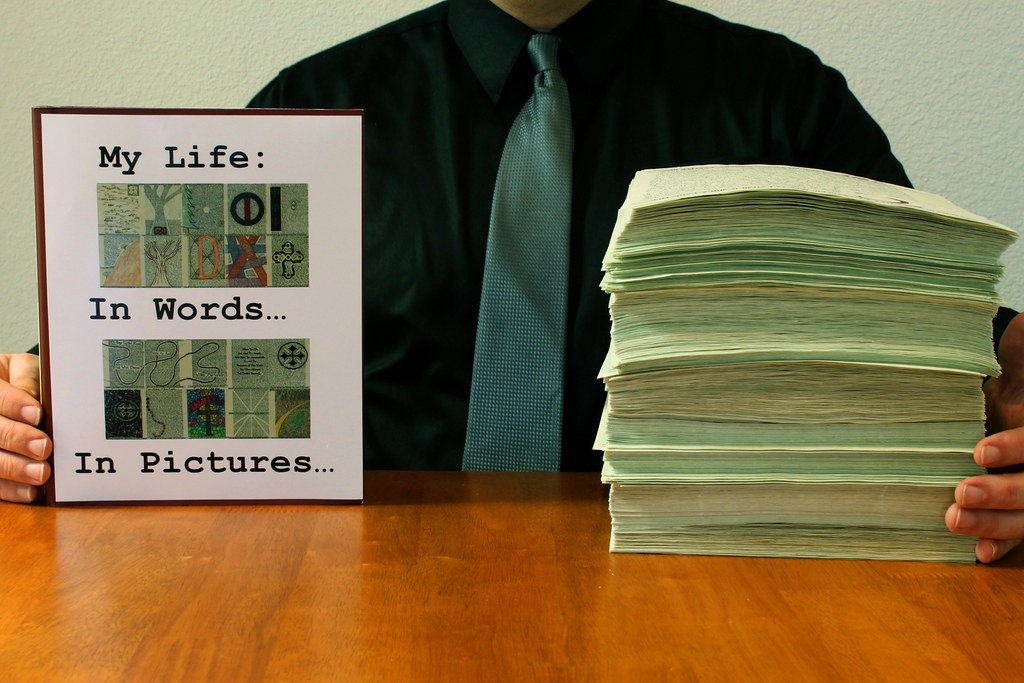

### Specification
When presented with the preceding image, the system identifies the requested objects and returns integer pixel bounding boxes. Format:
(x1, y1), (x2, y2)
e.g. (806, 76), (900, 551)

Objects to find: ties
(461, 37), (573, 473)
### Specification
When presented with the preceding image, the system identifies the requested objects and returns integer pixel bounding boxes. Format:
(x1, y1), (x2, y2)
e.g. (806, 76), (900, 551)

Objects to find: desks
(0, 468), (1024, 683)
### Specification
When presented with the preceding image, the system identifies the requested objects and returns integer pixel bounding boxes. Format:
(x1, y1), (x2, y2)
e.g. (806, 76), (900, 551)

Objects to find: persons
(1, 1), (1024, 563)
